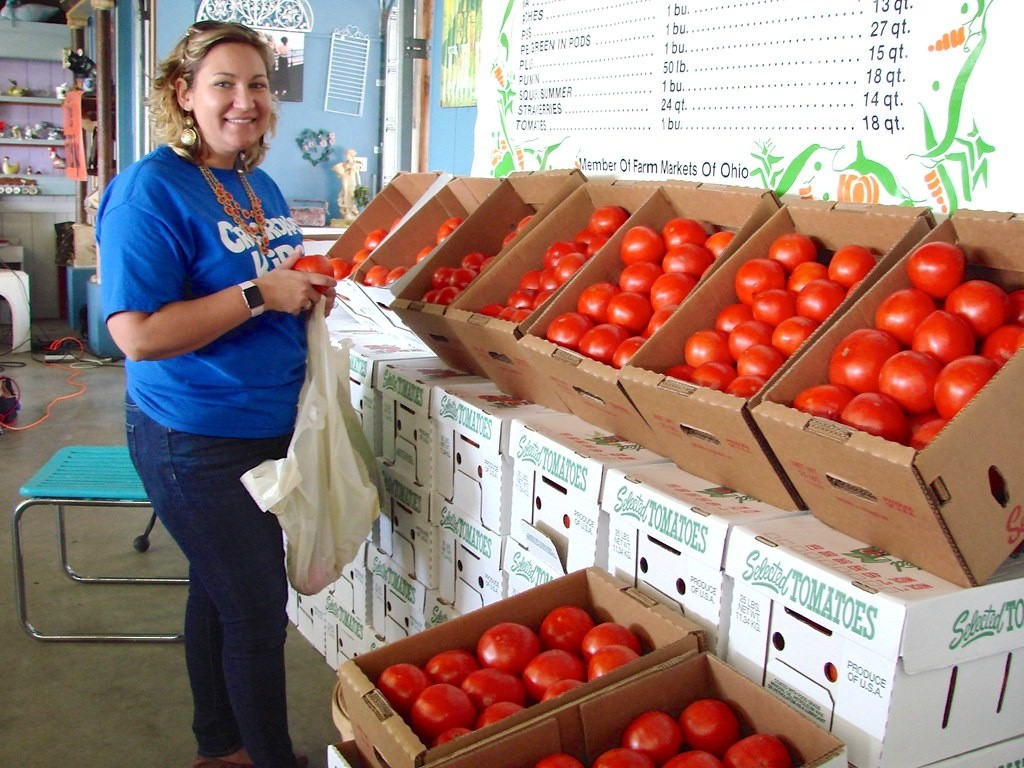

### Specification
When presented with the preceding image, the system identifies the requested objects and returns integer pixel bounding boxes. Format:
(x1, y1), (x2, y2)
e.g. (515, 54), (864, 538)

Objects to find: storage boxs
(284, 170), (1024, 768)
(71, 222), (100, 267)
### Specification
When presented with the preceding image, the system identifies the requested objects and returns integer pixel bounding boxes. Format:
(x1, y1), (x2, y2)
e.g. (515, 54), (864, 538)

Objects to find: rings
(306, 300), (312, 309)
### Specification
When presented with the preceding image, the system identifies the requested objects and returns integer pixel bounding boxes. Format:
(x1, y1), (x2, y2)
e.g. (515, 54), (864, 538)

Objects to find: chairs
(11, 444), (188, 646)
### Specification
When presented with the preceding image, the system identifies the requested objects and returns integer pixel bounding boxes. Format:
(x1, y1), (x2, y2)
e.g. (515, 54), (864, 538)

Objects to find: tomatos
(291, 205), (1024, 450)
(375, 604), (793, 768)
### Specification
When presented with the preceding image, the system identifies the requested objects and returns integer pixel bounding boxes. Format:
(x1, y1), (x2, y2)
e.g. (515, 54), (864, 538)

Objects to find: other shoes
(194, 745), (308, 768)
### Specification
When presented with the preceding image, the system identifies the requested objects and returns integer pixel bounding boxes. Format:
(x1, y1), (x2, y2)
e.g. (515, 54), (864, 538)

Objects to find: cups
(55, 87), (69, 99)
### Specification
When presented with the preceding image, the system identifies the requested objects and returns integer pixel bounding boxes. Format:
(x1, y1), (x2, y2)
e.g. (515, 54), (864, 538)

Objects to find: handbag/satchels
(240, 295), (384, 596)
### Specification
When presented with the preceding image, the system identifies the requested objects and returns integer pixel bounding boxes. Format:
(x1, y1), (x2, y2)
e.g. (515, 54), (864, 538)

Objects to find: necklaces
(198, 161), (270, 255)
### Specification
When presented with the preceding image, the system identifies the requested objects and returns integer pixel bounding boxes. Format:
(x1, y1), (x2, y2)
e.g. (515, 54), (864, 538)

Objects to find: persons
(94, 22), (337, 768)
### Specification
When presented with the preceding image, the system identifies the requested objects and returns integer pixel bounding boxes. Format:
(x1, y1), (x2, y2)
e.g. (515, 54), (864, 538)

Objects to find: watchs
(238, 281), (266, 318)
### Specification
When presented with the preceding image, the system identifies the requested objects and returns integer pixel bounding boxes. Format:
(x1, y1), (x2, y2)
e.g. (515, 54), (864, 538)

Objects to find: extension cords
(44, 354), (77, 363)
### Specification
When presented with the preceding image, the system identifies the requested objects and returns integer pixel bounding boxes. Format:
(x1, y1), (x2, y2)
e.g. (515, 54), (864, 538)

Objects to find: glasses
(180, 20), (260, 70)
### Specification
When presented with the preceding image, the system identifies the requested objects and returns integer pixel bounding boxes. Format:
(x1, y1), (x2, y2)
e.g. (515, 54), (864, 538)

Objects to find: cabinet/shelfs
(0, 16), (76, 325)
